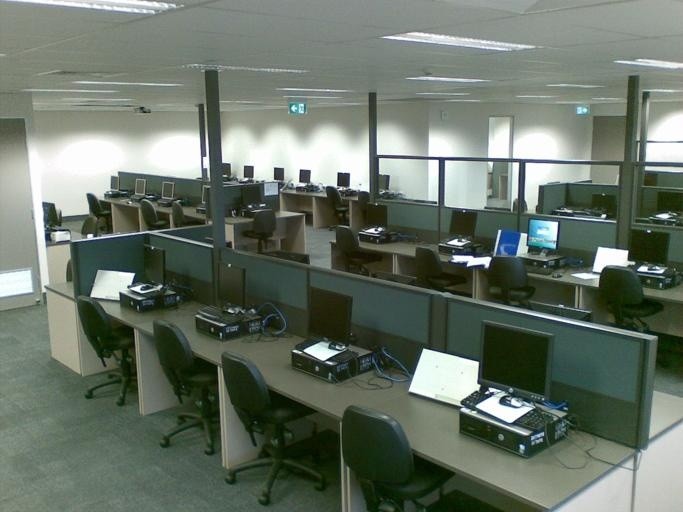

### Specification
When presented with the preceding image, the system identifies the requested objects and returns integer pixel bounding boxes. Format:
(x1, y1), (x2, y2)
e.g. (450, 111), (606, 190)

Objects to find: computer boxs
(195, 308), (262, 342)
(458, 408), (568, 457)
(119, 289), (178, 313)
(358, 231), (681, 290)
(291, 337), (373, 385)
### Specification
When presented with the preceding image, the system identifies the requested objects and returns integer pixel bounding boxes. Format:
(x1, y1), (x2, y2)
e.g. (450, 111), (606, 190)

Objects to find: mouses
(500, 394), (523, 408)
(328, 342), (343, 351)
(139, 284), (153, 291)
(223, 307), (237, 315)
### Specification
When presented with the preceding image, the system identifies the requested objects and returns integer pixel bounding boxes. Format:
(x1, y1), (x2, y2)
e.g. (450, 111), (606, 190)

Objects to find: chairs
(325, 187), (350, 225)
(244, 208), (278, 253)
(173, 201), (202, 227)
(152, 318), (218, 456)
(140, 198), (170, 230)
(75, 296), (141, 407)
(218, 346), (333, 506)
(358, 191), (369, 225)
(87, 193), (113, 234)
(340, 402), (457, 512)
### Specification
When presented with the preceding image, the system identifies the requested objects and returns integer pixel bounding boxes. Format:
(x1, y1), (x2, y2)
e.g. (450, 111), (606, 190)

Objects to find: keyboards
(295, 338), (359, 364)
(461, 390), (554, 430)
(128, 282), (165, 298)
(197, 305), (242, 325)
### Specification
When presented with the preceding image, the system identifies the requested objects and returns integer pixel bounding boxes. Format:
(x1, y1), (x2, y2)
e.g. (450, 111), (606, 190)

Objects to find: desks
(45, 230), (87, 285)
(35, 224), (683, 512)
(329, 227), (683, 338)
(633, 391), (683, 512)
(98, 193), (307, 266)
(43, 281), (81, 375)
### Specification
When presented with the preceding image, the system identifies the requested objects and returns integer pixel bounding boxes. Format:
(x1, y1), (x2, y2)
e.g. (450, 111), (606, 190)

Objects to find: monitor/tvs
(216, 262), (247, 310)
(476, 320), (555, 405)
(307, 284), (354, 348)
(374, 272), (414, 287)
(142, 242), (167, 285)
(517, 297), (593, 323)
(106, 161), (682, 268)
